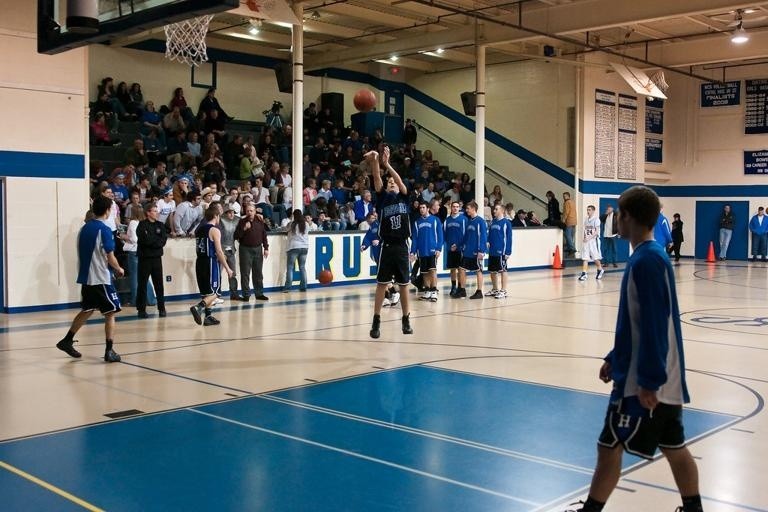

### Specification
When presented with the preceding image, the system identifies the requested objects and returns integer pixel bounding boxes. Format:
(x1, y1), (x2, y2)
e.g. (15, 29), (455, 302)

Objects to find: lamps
(729, 11), (754, 45)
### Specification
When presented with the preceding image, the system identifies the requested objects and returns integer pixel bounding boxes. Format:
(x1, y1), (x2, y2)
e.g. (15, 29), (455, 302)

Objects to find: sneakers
(568, 250), (574, 256)
(190, 304), (202, 325)
(204, 315), (220, 326)
(370, 318), (380, 338)
(579, 262), (619, 280)
(565, 500), (602, 512)
(138, 310), (149, 318)
(159, 309), (167, 317)
(212, 287), (307, 306)
(105, 349), (121, 362)
(57, 338), (81, 358)
(674, 506), (703, 512)
(381, 285), (508, 307)
(402, 313), (413, 334)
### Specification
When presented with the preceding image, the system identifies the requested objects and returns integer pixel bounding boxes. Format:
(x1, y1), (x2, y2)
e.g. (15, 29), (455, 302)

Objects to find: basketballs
(319, 271), (333, 284)
(354, 89), (376, 111)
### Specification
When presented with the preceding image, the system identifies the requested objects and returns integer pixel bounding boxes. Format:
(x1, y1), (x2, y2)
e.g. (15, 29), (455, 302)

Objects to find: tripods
(268, 111), (284, 128)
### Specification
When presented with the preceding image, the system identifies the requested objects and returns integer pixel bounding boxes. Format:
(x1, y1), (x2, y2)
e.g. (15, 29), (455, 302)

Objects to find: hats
(224, 206), (238, 214)
(111, 171), (125, 181)
(160, 184), (174, 197)
(200, 187), (215, 200)
(177, 175), (191, 182)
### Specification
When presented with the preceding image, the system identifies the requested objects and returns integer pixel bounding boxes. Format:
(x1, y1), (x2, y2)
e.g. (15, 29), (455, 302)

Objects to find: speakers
(460, 92), (476, 116)
(274, 63), (293, 94)
(321, 93), (344, 128)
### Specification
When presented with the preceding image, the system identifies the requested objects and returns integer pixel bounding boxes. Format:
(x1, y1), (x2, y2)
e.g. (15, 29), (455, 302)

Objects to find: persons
(598, 206), (619, 267)
(208, 201), (309, 300)
(99, 188), (170, 318)
(653, 205), (674, 254)
(667, 213), (684, 261)
(360, 198), (513, 307)
(89, 76), (576, 236)
(56, 196), (124, 362)
(718, 204), (735, 261)
(578, 205), (604, 281)
(362, 145), (412, 338)
(190, 206), (235, 326)
(564, 185), (703, 512)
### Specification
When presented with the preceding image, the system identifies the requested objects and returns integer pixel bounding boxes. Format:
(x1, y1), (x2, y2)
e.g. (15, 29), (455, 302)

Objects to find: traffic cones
(552, 244), (564, 269)
(704, 240), (717, 262)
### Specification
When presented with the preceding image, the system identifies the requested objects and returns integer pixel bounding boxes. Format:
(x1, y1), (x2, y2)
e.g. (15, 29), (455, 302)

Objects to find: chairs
(89, 101), (546, 231)
